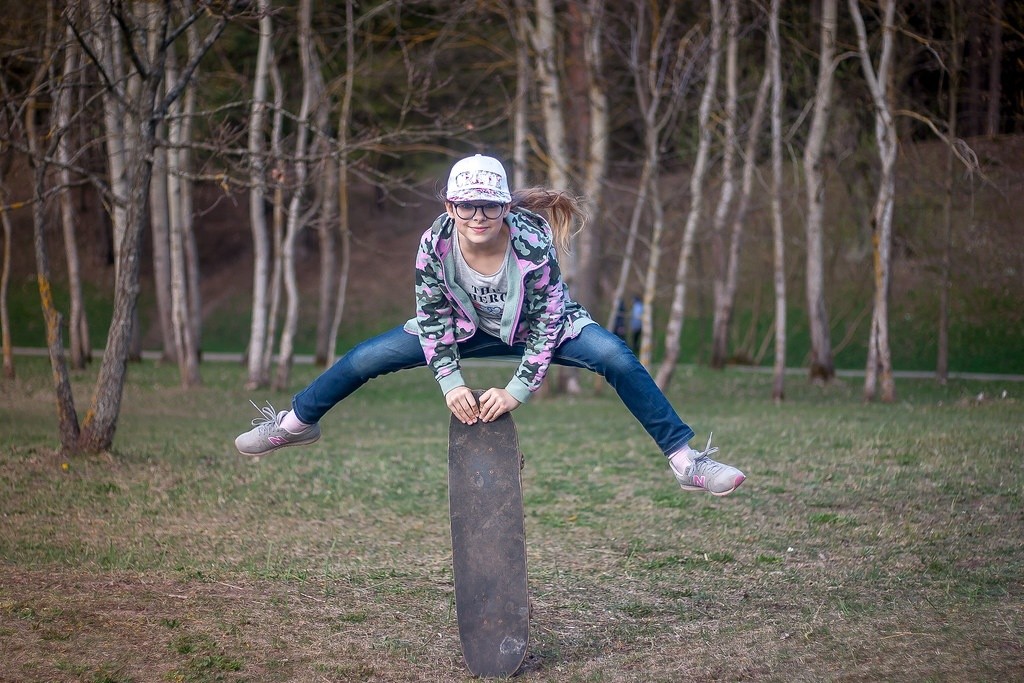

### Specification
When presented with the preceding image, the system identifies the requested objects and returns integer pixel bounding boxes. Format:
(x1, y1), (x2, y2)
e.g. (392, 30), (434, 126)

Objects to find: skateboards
(449, 387), (529, 680)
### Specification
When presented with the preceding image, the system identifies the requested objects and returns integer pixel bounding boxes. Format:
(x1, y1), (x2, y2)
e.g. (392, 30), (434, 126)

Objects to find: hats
(447, 153), (512, 205)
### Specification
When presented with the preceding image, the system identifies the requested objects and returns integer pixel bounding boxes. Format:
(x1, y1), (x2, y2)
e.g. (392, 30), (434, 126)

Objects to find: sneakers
(235, 399), (321, 456)
(669, 432), (746, 497)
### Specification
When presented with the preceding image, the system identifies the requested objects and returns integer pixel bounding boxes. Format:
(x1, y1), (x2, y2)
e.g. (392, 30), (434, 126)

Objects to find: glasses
(447, 200), (506, 220)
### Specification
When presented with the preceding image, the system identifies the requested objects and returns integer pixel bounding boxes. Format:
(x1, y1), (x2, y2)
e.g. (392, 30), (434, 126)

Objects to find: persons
(630, 293), (646, 348)
(233, 153), (746, 496)
(612, 292), (626, 339)
